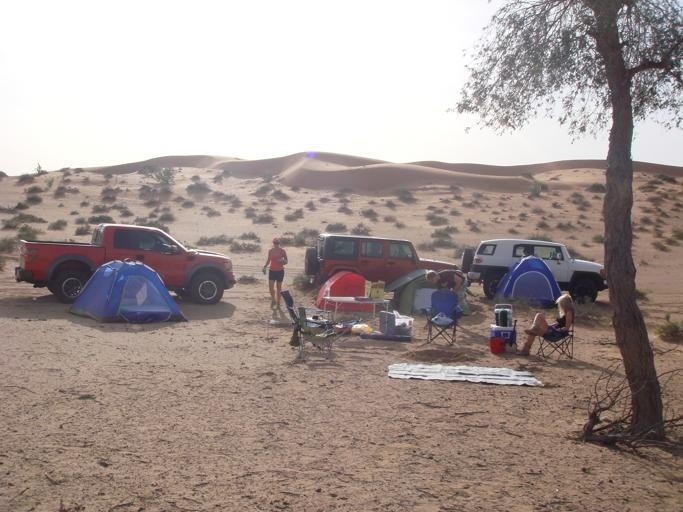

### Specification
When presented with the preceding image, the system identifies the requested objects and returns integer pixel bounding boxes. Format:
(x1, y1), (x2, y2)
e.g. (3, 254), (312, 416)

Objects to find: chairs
(420, 289), (463, 346)
(279, 289), (334, 361)
(536, 318), (575, 360)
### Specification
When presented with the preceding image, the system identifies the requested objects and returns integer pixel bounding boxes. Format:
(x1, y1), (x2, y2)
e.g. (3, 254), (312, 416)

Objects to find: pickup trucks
(14, 223), (235, 304)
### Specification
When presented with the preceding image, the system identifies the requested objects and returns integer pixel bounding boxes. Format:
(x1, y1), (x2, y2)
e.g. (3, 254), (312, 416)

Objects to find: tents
(491, 253), (564, 309)
(384, 268), (473, 317)
(313, 270), (385, 311)
(68, 258), (189, 325)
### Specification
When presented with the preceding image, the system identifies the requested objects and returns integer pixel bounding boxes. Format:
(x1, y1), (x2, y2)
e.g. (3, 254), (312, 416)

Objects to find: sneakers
(520, 344), (531, 355)
(524, 326), (538, 336)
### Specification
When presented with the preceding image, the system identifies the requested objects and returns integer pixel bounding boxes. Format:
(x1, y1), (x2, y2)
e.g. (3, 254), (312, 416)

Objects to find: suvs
(304, 235), (457, 287)
(460, 238), (609, 302)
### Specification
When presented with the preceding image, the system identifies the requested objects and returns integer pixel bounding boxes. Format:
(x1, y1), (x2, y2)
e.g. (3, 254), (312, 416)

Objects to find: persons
(262, 237), (288, 311)
(426, 269), (472, 316)
(514, 294), (576, 356)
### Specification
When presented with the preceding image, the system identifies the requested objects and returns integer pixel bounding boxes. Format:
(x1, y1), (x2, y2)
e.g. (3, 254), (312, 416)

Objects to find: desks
(322, 295), (391, 333)
